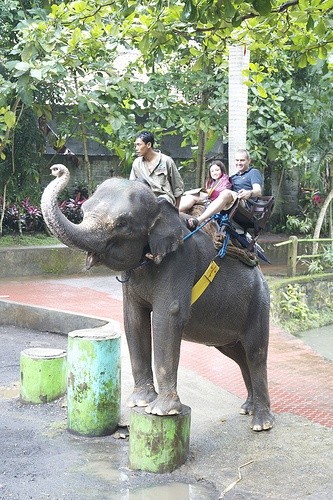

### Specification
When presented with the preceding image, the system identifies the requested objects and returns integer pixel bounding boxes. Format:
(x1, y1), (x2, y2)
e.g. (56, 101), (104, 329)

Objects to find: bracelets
(249, 191), (253, 198)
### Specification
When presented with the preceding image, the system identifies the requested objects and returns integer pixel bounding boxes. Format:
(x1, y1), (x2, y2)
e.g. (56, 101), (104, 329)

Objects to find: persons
(179, 160), (233, 212)
(185, 149), (262, 232)
(129, 130), (185, 209)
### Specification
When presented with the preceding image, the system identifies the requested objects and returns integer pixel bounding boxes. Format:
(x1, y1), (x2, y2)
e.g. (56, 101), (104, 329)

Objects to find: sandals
(185, 217), (200, 232)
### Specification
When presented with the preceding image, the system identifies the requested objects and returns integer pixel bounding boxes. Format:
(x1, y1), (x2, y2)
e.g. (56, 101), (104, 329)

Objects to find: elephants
(41, 163), (275, 432)
